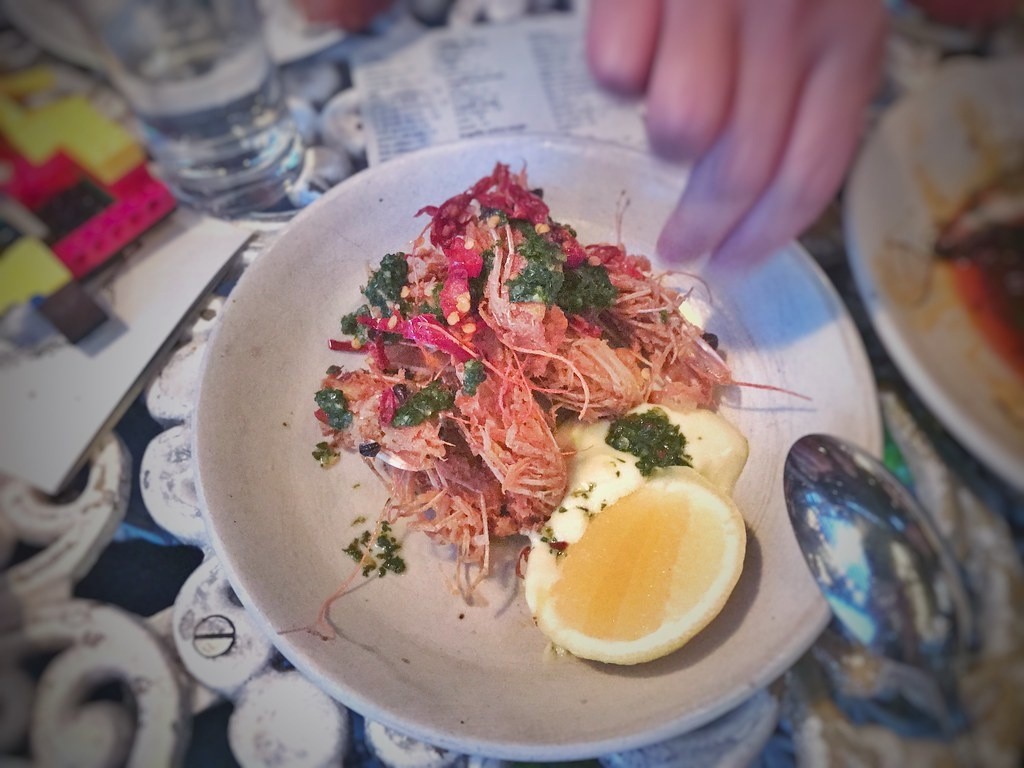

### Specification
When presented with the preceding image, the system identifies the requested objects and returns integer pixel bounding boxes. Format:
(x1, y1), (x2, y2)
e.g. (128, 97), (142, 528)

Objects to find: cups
(85, 1), (302, 218)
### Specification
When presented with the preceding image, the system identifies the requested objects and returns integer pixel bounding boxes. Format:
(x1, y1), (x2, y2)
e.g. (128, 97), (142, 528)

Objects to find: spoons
(786, 434), (974, 738)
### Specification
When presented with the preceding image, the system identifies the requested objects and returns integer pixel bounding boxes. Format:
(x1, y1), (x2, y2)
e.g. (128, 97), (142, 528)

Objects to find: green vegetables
(312, 209), (695, 580)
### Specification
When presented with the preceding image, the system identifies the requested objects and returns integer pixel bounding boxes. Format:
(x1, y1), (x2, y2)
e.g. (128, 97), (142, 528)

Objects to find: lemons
(536, 463), (748, 665)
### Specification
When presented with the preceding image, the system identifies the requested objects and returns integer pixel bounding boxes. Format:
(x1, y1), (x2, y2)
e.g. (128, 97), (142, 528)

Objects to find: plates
(192, 134), (885, 762)
(6, 0), (349, 70)
(846, 58), (1024, 489)
